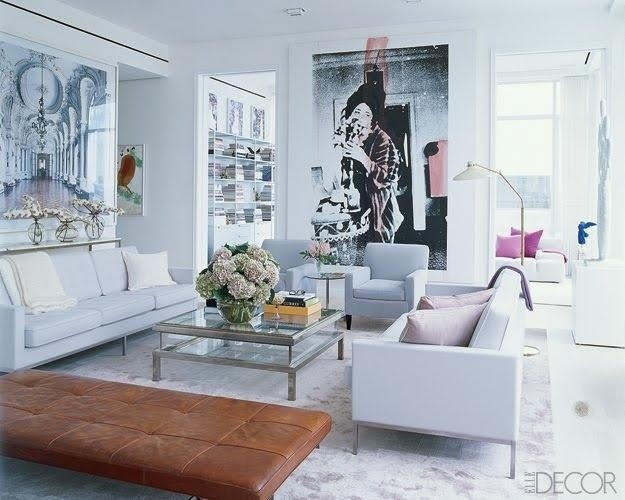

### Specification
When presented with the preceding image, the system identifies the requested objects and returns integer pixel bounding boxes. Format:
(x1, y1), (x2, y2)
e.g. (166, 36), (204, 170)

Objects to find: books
(264, 290), (322, 328)
(208, 135), (276, 224)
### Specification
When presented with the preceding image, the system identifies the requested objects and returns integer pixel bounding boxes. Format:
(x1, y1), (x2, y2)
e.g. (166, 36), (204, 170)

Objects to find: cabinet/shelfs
(209, 130), (275, 260)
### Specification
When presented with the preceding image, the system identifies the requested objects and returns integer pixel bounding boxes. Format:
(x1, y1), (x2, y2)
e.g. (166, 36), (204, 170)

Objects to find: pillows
(90, 249), (177, 296)
(401, 288), (493, 347)
(496, 227), (544, 257)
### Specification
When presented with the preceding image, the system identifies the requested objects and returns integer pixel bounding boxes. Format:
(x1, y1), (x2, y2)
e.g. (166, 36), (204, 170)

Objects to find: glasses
(289, 290), (305, 295)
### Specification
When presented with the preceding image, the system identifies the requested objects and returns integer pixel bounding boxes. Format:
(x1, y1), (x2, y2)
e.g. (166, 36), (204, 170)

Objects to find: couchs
(0, 246), (206, 373)
(494, 239), (568, 281)
(352, 263), (526, 478)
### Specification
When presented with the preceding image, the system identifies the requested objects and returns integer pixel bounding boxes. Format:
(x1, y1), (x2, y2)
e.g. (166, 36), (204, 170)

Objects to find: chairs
(262, 239), (319, 292)
(344, 243), (429, 330)
(572, 257), (624, 348)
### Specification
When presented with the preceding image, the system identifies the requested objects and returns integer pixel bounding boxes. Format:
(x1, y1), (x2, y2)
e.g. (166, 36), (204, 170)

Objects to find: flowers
(3, 193), (123, 221)
(196, 243), (280, 321)
(300, 238), (337, 265)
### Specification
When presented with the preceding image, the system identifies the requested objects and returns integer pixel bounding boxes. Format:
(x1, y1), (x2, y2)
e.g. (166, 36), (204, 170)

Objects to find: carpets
(0, 323), (553, 499)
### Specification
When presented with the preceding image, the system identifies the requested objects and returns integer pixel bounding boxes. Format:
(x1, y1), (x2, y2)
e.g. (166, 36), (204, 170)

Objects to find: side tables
(305, 273), (345, 309)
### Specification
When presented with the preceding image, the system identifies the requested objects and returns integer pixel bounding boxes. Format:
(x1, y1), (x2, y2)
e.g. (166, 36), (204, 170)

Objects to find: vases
(315, 261), (321, 274)
(28, 220), (103, 244)
(217, 297), (261, 324)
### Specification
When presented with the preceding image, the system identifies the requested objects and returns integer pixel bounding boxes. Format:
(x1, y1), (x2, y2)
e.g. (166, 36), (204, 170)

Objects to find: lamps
(454, 161), (524, 265)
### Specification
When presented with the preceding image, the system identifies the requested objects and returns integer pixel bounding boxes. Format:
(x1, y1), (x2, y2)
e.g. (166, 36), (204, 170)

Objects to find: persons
(340, 84), (405, 244)
(39, 159), (46, 179)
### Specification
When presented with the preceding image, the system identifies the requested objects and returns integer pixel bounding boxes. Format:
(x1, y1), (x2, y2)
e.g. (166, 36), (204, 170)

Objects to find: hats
(345, 83), (379, 126)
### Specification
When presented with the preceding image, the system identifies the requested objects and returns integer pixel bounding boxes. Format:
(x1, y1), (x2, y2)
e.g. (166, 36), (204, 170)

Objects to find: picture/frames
(118, 143), (145, 216)
(1, 30), (118, 234)
(208, 91), (266, 140)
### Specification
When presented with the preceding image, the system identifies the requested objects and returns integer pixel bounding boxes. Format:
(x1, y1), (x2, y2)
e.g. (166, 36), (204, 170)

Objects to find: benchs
(0, 369), (333, 499)
